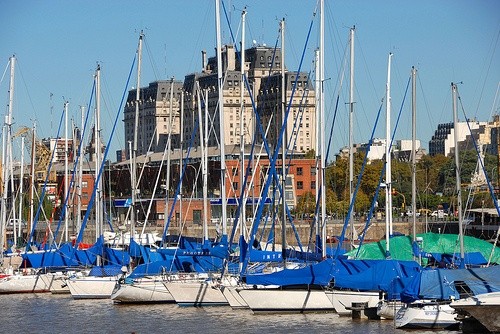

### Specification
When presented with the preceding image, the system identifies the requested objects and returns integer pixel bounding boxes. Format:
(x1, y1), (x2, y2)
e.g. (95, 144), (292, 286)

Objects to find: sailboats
(0, 0), (500, 333)
